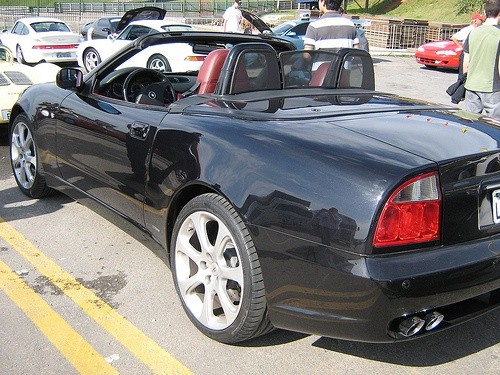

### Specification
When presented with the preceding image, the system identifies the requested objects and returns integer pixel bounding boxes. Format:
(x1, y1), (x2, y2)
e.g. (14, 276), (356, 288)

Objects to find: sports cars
(8, 30), (499, 345)
(414, 25), (476, 73)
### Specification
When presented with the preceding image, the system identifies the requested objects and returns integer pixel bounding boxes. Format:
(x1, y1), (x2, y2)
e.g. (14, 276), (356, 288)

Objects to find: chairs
(22, 24), (36, 34)
(176, 48), (252, 101)
(48, 24), (58, 31)
(308, 61), (351, 89)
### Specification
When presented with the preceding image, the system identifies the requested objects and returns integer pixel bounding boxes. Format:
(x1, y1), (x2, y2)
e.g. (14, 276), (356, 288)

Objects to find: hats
(471, 13), (485, 20)
(235, 0), (242, 5)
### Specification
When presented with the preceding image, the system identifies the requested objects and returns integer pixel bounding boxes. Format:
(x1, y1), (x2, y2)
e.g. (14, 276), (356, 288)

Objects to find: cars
(240, 9), (370, 66)
(0, 6), (227, 125)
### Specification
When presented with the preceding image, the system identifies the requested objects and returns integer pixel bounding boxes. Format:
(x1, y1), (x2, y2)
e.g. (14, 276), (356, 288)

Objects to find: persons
(452, 14), (486, 74)
(222, 0), (243, 33)
(463, 0), (500, 122)
(302, 0), (360, 87)
